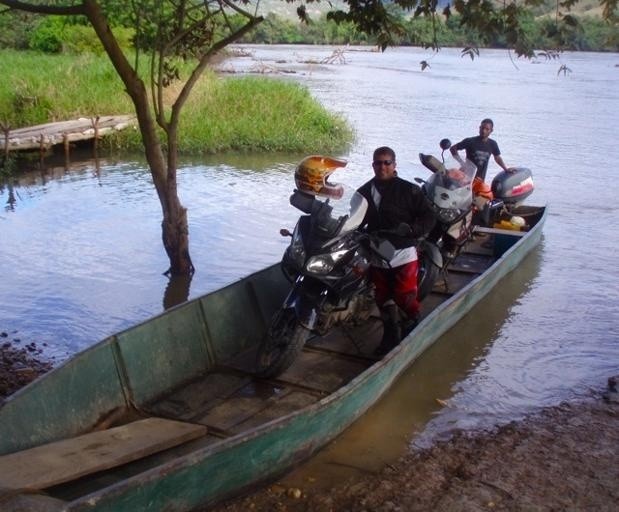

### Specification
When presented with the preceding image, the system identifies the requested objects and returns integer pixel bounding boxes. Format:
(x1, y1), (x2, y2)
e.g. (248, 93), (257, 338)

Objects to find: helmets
(294, 155), (348, 200)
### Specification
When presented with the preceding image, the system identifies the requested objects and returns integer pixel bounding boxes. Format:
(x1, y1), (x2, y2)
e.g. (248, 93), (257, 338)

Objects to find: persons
(449, 117), (517, 243)
(344, 147), (436, 358)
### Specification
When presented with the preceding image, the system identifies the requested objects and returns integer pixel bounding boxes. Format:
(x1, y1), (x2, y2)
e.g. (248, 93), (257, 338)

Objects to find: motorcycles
(414, 138), (477, 303)
(255, 183), (409, 382)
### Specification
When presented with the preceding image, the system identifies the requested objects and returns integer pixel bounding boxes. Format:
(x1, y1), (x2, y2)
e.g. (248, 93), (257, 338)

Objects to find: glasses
(374, 160), (397, 165)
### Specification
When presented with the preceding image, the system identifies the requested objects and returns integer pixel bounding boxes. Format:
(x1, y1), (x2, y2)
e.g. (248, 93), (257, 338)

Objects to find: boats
(0, 167), (549, 512)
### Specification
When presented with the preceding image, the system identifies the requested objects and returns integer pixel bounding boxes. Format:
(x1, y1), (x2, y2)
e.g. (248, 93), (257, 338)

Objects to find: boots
(373, 306), (400, 356)
